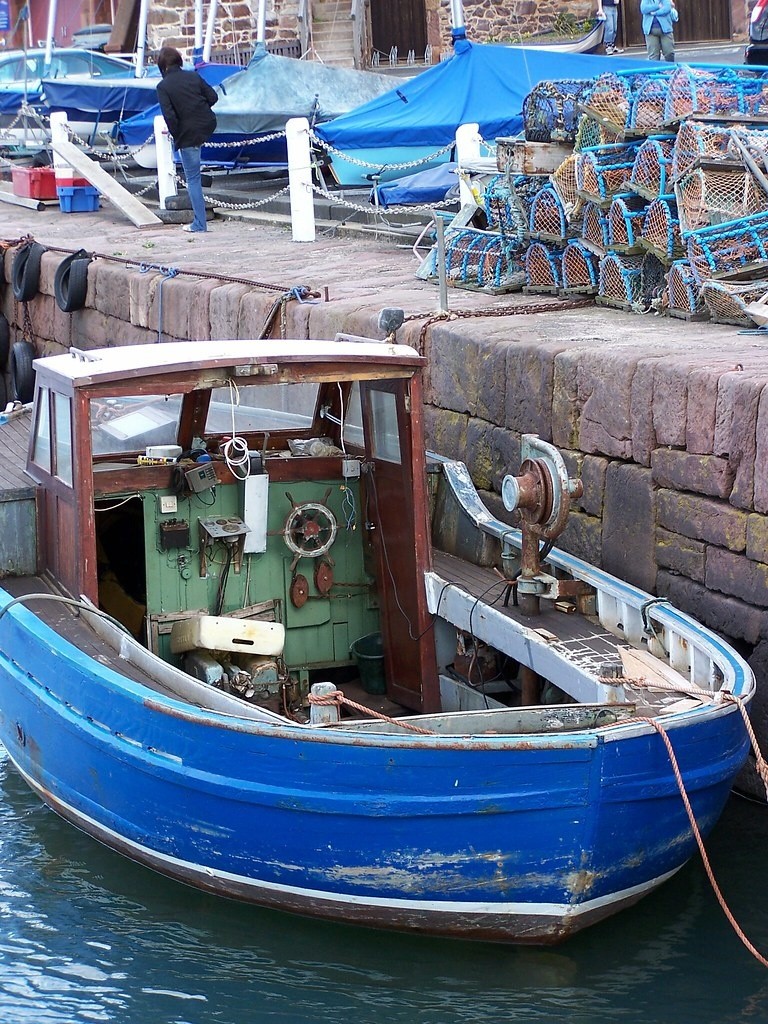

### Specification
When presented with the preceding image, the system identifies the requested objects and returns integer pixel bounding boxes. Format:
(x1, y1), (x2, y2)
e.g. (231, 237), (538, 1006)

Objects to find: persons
(640, 0), (679, 62)
(156, 46), (219, 233)
(596, 0), (625, 55)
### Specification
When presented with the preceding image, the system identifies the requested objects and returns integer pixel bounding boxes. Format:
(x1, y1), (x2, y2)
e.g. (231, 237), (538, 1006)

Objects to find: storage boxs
(495, 137), (573, 174)
(55, 177), (92, 187)
(56, 186), (101, 213)
(55, 162), (99, 178)
(10, 168), (59, 199)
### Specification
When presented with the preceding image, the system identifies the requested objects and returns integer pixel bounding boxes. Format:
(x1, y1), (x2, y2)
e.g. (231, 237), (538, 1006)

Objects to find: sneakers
(606, 46), (613, 55)
(613, 46), (624, 53)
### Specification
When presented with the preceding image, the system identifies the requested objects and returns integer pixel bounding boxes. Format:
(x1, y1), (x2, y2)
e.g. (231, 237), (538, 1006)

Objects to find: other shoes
(183, 223), (206, 231)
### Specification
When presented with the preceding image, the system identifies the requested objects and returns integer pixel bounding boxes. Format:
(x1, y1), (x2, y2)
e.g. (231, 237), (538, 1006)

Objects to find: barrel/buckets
(349, 631), (386, 695)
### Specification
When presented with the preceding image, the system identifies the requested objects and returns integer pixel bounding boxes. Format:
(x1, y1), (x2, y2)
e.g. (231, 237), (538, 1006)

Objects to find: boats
(2, 332), (759, 939)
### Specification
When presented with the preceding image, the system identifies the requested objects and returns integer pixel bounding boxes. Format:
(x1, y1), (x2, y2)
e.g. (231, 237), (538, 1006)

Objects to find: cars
(0, 47), (163, 89)
(740, 0), (768, 65)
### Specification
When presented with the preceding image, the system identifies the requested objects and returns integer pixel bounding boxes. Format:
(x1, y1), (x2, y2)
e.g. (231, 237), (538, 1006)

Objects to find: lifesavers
(0, 374), (7, 412)
(11, 242), (44, 301)
(53, 253), (91, 313)
(0, 314), (10, 371)
(10, 342), (36, 404)
